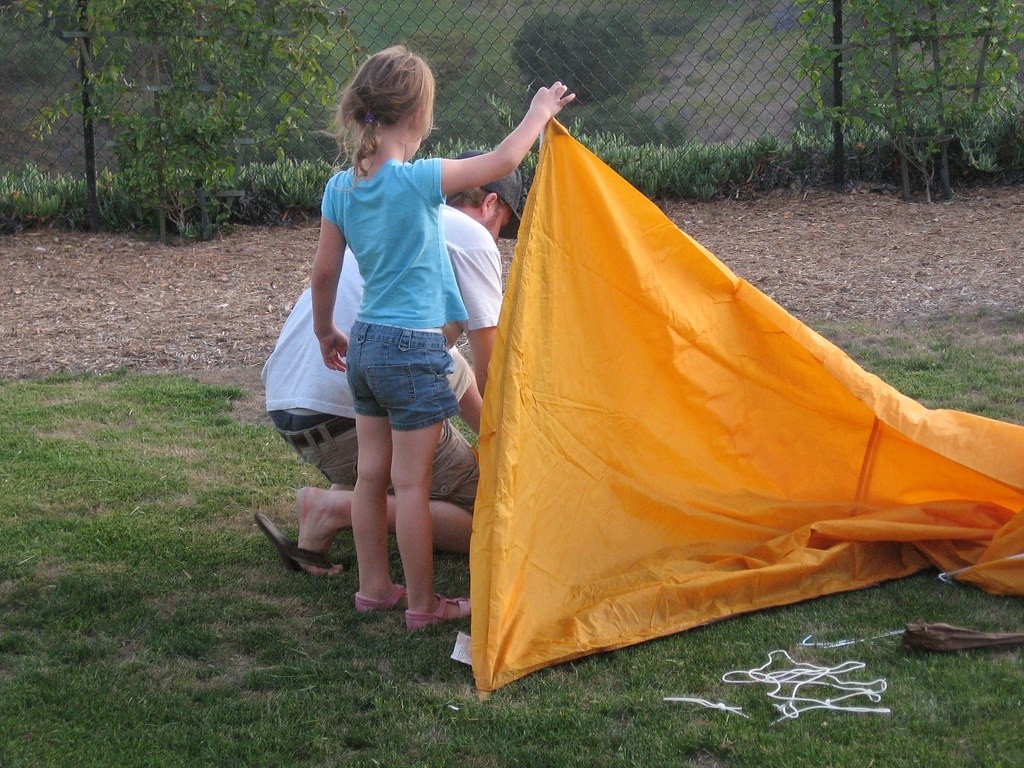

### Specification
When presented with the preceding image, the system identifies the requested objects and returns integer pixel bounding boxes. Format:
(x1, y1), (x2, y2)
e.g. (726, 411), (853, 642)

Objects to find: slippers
(255, 512), (344, 575)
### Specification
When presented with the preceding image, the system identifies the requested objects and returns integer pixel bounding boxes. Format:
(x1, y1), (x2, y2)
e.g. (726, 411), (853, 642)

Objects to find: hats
(453, 151), (523, 239)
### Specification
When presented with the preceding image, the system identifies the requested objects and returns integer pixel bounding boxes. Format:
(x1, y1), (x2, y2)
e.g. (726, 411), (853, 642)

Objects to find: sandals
(405, 593), (472, 630)
(354, 584), (408, 612)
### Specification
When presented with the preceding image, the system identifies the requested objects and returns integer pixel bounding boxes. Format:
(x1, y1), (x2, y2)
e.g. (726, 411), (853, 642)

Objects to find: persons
(252, 150), (523, 577)
(311, 46), (576, 632)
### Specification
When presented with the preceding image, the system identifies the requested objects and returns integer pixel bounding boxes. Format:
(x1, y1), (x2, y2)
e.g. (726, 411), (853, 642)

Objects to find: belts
(275, 417), (355, 445)
